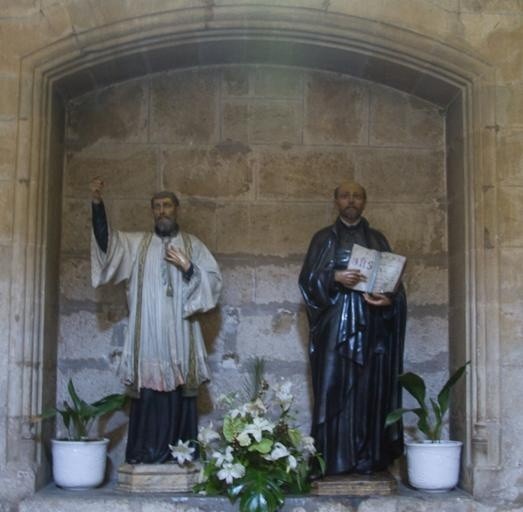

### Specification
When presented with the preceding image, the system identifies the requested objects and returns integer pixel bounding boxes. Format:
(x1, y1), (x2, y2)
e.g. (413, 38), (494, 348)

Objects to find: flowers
(166, 356), (327, 512)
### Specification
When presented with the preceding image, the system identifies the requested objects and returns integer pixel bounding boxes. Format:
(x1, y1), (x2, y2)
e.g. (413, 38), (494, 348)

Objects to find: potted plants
(32, 378), (127, 492)
(384, 359), (472, 492)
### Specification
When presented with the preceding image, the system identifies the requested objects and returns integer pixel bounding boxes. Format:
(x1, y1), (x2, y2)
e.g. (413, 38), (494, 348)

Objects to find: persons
(88, 178), (223, 464)
(298, 181), (407, 483)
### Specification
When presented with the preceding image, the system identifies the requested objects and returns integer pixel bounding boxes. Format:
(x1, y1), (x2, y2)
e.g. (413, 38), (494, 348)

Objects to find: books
(344, 243), (406, 296)
